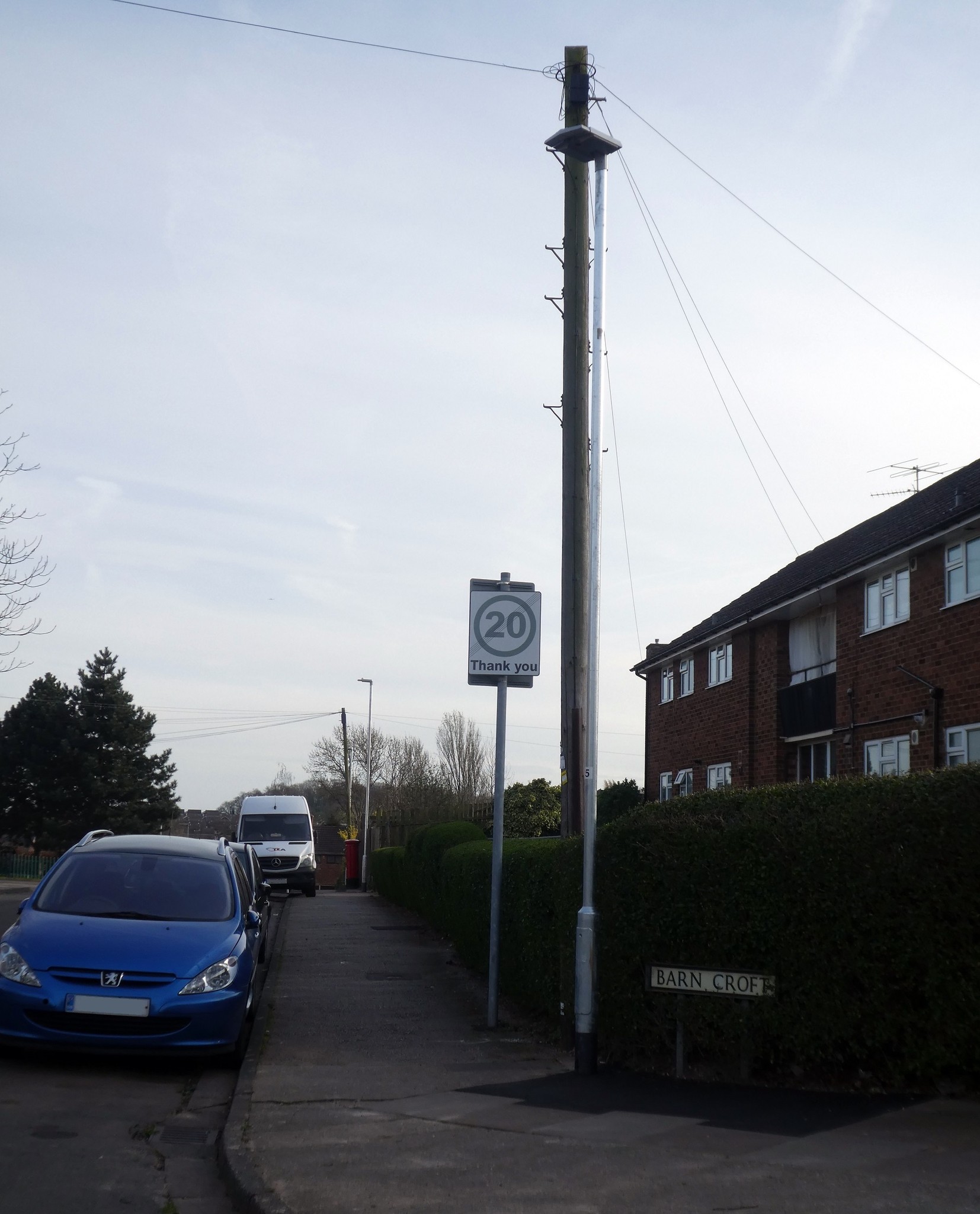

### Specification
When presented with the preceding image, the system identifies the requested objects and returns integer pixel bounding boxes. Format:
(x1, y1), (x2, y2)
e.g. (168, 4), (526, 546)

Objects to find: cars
(0, 829), (274, 1075)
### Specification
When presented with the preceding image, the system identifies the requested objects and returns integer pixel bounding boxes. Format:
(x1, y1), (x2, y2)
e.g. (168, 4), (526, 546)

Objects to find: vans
(231, 794), (319, 897)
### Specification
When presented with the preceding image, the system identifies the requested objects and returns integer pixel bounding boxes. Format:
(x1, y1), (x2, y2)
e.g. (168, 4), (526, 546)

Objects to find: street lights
(358, 677), (374, 893)
